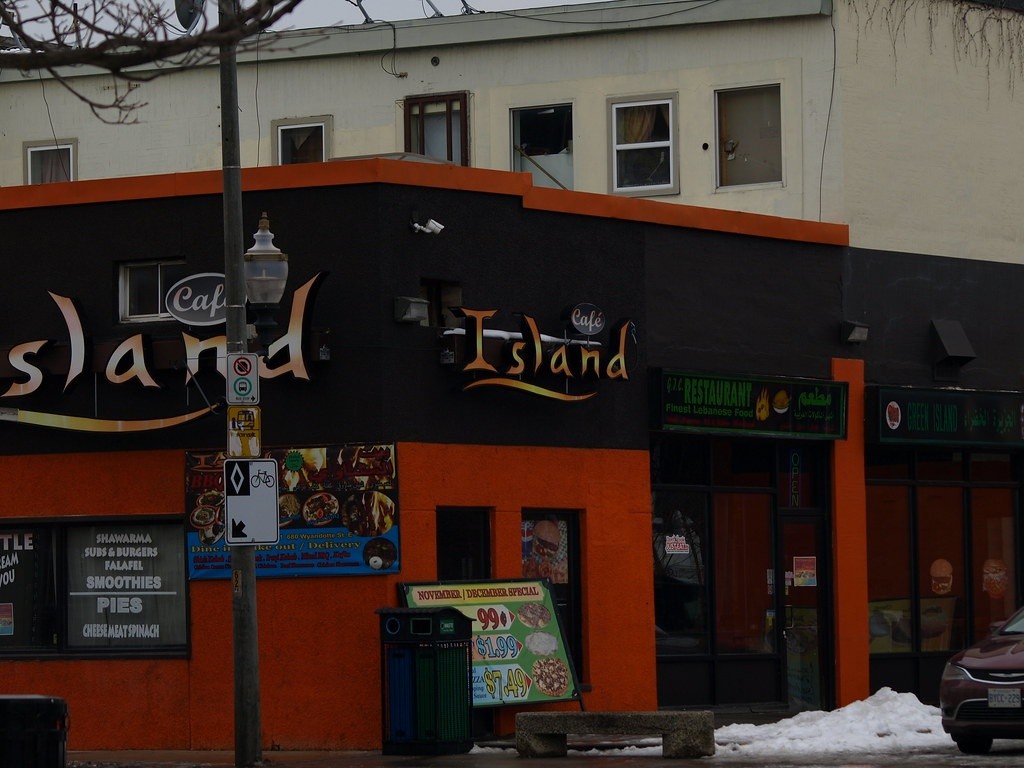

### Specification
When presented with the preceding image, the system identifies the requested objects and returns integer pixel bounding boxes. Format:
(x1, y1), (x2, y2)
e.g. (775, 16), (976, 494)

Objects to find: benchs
(513, 710), (715, 760)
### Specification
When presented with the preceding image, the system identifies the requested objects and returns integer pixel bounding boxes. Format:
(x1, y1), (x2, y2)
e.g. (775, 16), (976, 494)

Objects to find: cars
(940, 605), (1024, 753)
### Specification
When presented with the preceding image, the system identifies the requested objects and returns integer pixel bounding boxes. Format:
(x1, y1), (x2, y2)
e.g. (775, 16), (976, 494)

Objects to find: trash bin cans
(0, 693), (71, 768)
(375, 605), (478, 757)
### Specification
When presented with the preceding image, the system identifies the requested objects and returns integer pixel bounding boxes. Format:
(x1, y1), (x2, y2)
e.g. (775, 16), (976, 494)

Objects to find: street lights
(224, 213), (290, 768)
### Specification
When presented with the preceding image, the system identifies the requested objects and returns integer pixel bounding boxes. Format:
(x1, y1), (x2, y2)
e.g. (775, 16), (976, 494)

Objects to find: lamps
(244, 211), (289, 356)
(393, 296), (430, 325)
(840, 320), (871, 344)
